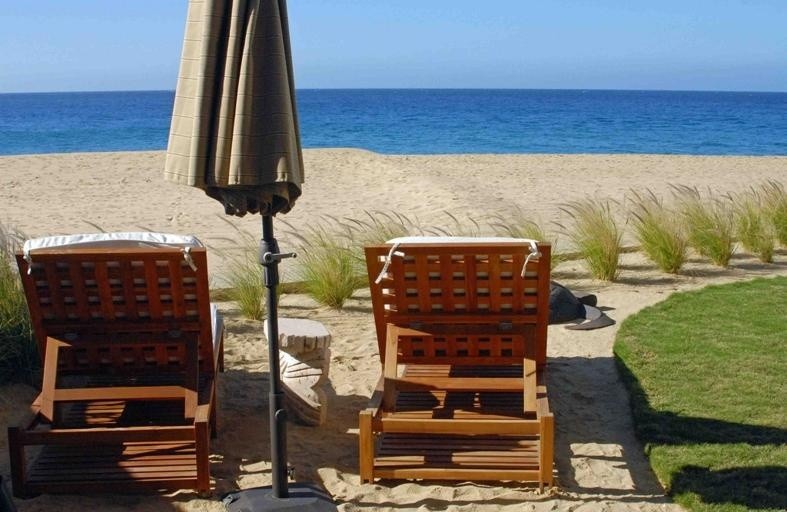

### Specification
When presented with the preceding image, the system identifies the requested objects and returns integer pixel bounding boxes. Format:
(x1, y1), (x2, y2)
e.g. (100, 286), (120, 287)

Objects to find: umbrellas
(164, 1), (306, 499)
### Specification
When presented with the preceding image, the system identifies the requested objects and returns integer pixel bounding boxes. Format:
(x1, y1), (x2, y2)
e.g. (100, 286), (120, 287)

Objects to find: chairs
(360, 245), (552, 492)
(7, 250), (224, 499)
